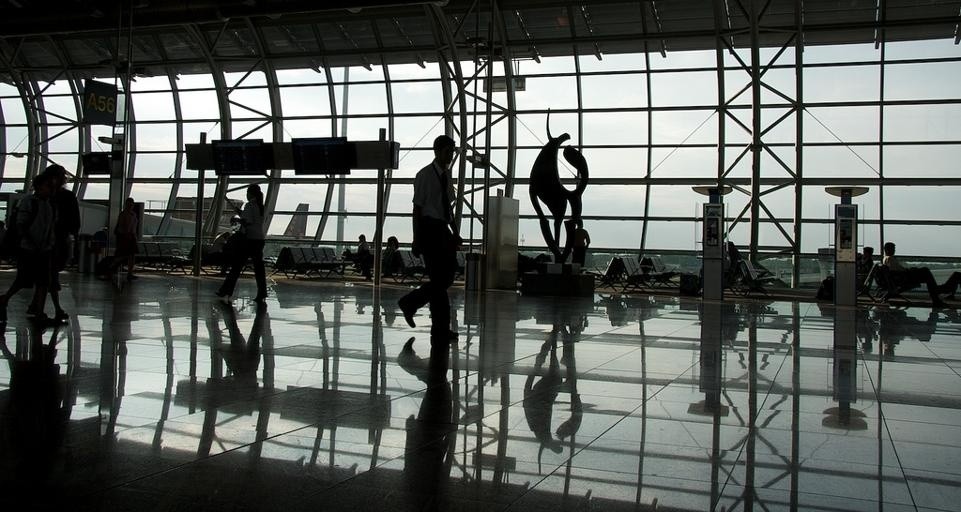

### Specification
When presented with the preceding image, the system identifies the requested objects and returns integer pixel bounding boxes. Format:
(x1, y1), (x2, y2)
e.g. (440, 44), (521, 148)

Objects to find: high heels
(216, 290), (233, 299)
(252, 294), (268, 302)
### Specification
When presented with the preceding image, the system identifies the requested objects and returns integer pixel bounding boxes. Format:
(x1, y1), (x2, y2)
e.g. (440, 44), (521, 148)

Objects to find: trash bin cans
(465, 252), (486, 291)
(680, 275), (697, 296)
(78, 235), (109, 273)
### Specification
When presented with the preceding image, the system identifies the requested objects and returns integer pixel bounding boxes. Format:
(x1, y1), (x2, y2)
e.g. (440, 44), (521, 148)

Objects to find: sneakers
(398, 337), (413, 358)
(398, 300), (415, 327)
(26, 307), (47, 318)
(54, 312), (68, 319)
(1, 295), (7, 322)
(430, 330), (459, 337)
(127, 275), (138, 280)
(103, 273), (112, 277)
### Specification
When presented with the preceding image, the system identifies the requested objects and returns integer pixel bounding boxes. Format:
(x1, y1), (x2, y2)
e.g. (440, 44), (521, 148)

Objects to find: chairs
(128, 240), (912, 306)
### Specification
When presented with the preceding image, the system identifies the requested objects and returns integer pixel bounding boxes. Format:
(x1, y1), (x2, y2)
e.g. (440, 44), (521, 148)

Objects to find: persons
(214, 300), (266, 387)
(105, 197), (140, 281)
(393, 336), (456, 512)
(882, 242), (946, 308)
(0, 176), (39, 322)
(851, 306), (938, 355)
(213, 182), (268, 305)
(856, 246), (875, 291)
(379, 235), (400, 277)
(398, 134), (463, 340)
(935, 271), (960, 300)
(570, 219), (589, 268)
(356, 234), (373, 280)
(21, 163), (80, 321)
